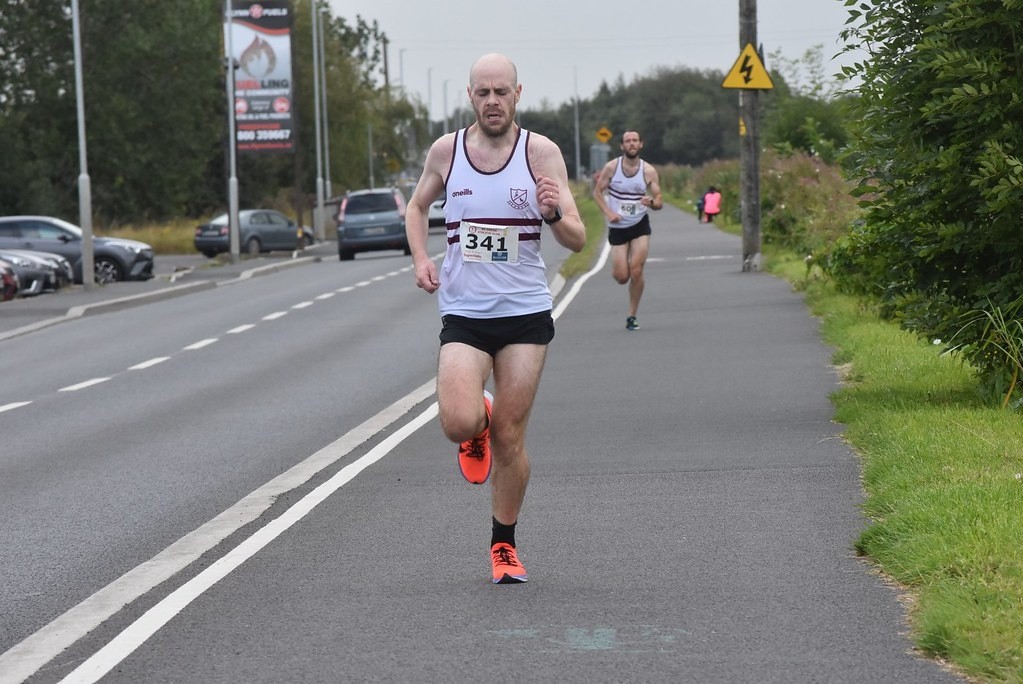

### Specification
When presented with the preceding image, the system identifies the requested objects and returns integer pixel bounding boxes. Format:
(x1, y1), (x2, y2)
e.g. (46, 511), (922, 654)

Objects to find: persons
(405, 54), (586, 584)
(697, 185), (722, 223)
(593, 130), (662, 330)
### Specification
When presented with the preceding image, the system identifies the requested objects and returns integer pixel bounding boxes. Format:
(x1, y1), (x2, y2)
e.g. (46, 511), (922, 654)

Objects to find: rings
(550, 192), (552, 197)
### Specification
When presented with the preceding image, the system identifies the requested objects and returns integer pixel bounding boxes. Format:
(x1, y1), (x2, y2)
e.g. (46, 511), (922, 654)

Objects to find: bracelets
(651, 200), (653, 206)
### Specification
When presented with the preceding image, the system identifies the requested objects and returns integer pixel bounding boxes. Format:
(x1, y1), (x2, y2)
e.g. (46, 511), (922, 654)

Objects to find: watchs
(541, 206), (563, 225)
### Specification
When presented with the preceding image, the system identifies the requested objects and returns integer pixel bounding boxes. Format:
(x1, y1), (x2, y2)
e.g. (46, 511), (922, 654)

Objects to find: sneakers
(625, 316), (640, 331)
(457, 389), (495, 485)
(490, 542), (528, 584)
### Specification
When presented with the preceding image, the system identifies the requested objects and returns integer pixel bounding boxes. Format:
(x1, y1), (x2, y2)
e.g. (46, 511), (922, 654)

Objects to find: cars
(193, 209), (316, 259)
(0, 215), (156, 285)
(0, 247), (75, 300)
(428, 191), (446, 225)
(0, 259), (19, 304)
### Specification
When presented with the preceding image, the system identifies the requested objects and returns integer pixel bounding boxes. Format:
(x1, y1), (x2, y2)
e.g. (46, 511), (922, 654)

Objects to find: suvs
(337, 185), (411, 262)
